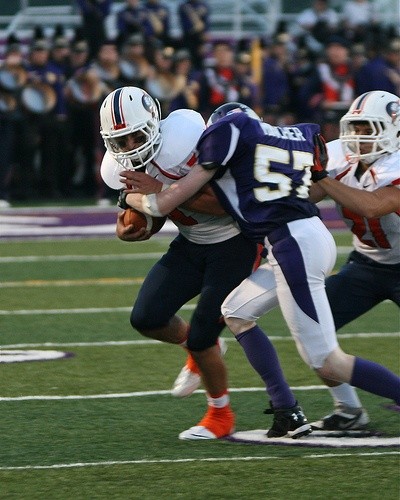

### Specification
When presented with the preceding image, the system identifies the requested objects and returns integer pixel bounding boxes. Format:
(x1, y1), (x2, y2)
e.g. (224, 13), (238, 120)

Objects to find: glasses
(117, 135), (146, 148)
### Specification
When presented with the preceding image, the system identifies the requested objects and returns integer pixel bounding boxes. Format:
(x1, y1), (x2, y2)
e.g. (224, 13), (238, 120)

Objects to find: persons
(99, 86), (264, 442)
(305, 91), (400, 437)
(0, 0), (400, 195)
(118, 102), (400, 438)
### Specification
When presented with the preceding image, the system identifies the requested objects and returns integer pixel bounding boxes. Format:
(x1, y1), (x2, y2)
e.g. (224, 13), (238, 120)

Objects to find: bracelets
(144, 214), (153, 232)
(161, 183), (169, 192)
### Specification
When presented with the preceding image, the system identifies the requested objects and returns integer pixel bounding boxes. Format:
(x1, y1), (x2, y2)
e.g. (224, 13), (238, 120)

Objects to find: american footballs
(123, 207), (167, 234)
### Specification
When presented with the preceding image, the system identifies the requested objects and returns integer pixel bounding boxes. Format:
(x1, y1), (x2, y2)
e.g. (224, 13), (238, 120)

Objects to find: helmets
(205, 101), (261, 128)
(99, 86), (160, 161)
(340, 90), (400, 153)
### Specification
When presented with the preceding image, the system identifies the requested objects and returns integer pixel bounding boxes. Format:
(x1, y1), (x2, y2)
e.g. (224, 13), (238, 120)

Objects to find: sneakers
(178, 400), (235, 441)
(171, 336), (228, 397)
(263, 401), (312, 438)
(310, 404), (371, 430)
